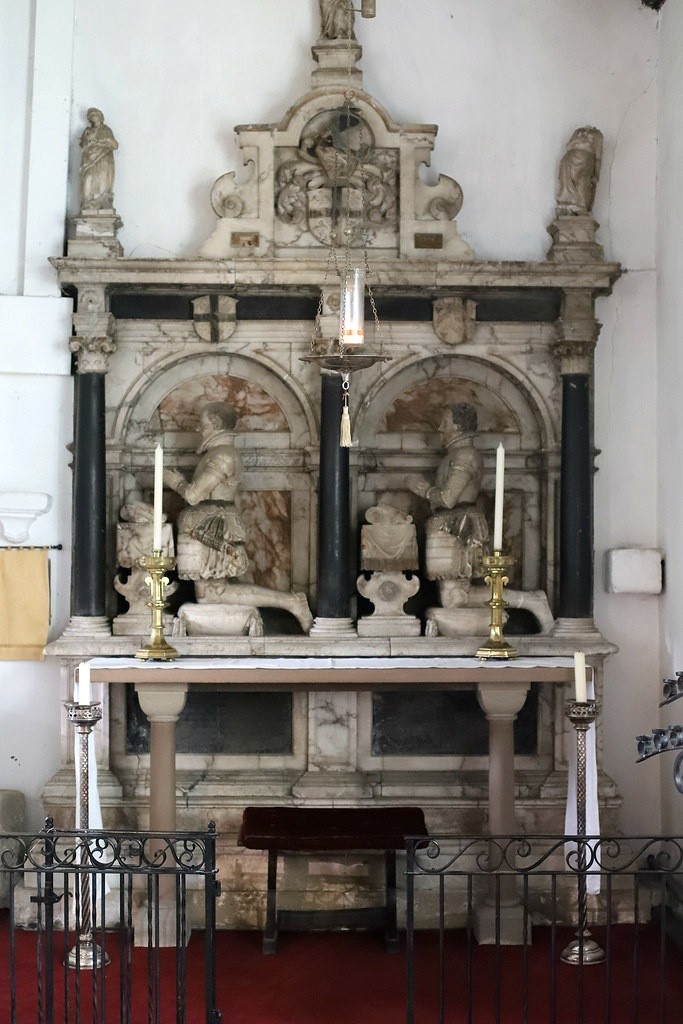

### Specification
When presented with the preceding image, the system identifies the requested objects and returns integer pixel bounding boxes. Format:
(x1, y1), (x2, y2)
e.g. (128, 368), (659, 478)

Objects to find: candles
(151, 439), (165, 551)
(574, 650), (587, 703)
(79, 658), (89, 705)
(492, 442), (506, 550)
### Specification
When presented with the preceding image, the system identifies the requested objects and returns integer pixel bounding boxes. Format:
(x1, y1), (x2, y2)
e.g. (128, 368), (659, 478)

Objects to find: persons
(404, 403), (555, 636)
(163, 402), (314, 634)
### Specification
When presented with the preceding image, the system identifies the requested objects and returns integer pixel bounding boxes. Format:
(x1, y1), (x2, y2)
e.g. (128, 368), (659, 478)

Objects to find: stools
(236, 806), (429, 955)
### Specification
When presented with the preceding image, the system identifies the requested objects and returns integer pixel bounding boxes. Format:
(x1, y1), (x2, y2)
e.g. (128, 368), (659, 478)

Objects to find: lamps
(297, 1), (394, 448)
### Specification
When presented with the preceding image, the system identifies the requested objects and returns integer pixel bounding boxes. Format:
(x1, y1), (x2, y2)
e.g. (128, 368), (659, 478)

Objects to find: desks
(71, 657), (601, 943)
